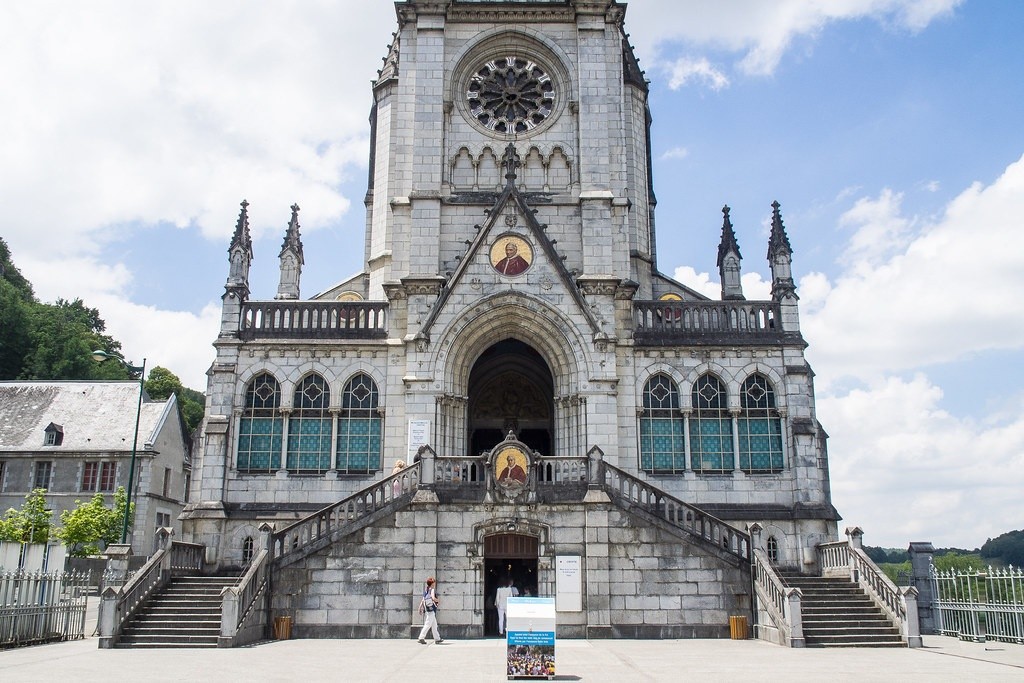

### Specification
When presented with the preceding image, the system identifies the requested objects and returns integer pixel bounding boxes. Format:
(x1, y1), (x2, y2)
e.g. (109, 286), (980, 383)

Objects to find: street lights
(91, 347), (147, 544)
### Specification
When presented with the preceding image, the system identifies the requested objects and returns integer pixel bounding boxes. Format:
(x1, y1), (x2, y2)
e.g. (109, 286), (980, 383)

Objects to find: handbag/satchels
(425, 603), (437, 611)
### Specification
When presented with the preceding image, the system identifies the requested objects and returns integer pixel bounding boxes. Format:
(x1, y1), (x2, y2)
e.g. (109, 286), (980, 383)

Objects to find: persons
(454, 454), (467, 482)
(416, 576), (444, 645)
(391, 459), (407, 500)
(507, 645), (554, 677)
(414, 445), (426, 476)
(487, 577), (532, 636)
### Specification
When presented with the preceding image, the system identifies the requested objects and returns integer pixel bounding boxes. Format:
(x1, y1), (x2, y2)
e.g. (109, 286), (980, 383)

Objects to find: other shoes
(435, 639), (443, 644)
(418, 639), (426, 644)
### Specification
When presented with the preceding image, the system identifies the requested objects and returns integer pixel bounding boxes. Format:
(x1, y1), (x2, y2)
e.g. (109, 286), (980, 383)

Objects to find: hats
(427, 578), (436, 585)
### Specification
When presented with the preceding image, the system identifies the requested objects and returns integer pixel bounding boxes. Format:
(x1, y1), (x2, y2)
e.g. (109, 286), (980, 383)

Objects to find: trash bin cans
(274, 615), (292, 640)
(731, 616), (749, 640)
